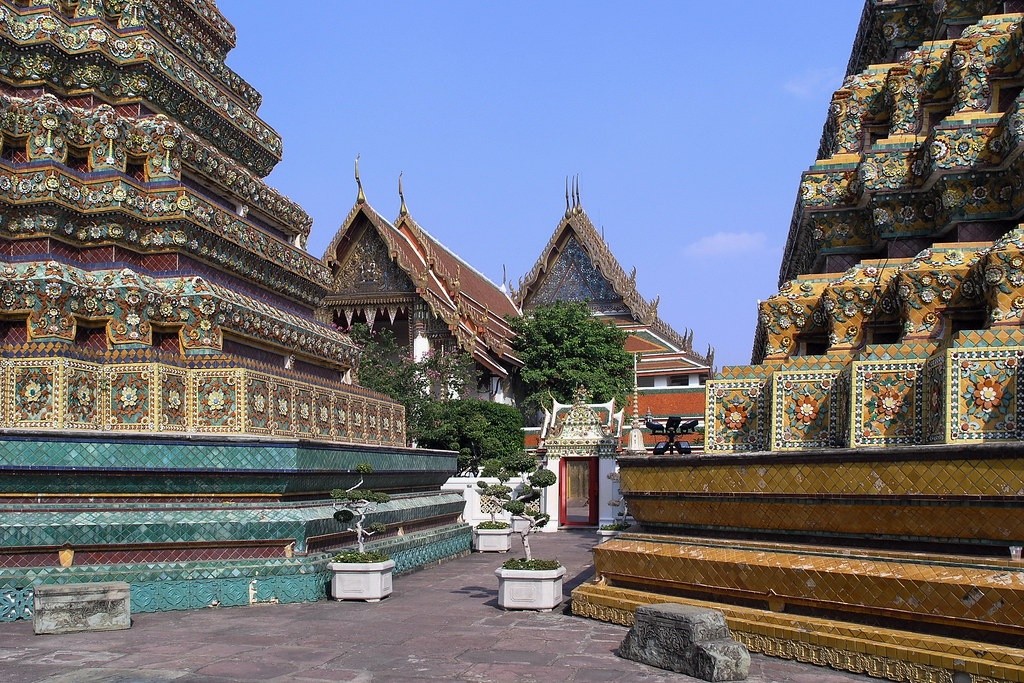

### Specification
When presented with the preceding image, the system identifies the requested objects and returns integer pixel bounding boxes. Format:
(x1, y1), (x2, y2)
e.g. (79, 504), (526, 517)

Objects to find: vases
(473, 528), (514, 554)
(494, 565), (565, 612)
(327, 559), (395, 603)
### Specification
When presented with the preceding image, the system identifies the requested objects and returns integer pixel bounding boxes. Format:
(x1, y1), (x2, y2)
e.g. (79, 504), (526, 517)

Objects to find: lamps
(646, 415), (697, 454)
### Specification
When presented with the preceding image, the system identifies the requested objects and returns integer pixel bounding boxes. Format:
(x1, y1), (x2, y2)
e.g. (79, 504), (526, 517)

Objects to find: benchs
(32, 582), (132, 636)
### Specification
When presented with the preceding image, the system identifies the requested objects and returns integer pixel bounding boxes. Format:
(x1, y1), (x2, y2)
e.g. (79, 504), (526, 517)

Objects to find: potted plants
(595, 472), (629, 545)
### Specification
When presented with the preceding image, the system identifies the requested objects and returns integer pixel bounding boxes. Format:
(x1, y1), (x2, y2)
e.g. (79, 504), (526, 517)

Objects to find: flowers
(498, 466), (561, 571)
(324, 465), (391, 564)
(475, 452), (536, 529)
